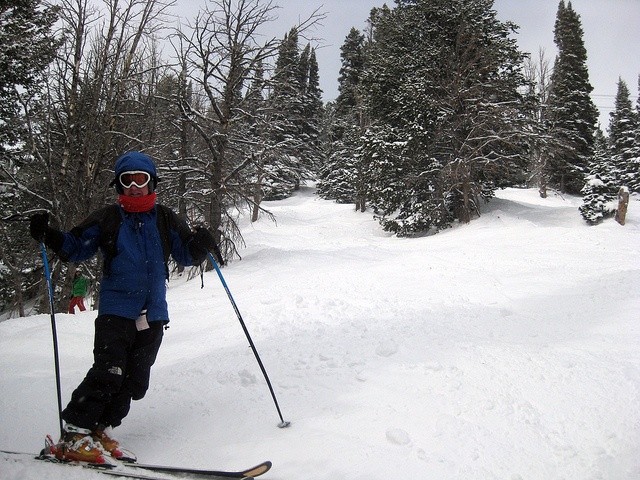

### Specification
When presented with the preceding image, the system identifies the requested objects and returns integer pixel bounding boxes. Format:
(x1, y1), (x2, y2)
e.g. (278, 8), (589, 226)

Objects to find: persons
(69, 270), (91, 314)
(30, 152), (214, 466)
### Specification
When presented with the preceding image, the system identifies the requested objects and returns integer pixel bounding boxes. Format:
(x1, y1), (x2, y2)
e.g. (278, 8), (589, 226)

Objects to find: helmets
(115, 152), (157, 194)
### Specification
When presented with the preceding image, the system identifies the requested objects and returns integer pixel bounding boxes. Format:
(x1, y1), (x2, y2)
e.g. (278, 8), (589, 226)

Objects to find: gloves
(189, 226), (216, 258)
(29, 212), (66, 255)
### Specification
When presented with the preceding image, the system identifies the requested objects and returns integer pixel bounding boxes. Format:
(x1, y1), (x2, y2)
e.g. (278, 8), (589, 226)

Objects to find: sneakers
(57, 432), (102, 460)
(89, 429), (119, 452)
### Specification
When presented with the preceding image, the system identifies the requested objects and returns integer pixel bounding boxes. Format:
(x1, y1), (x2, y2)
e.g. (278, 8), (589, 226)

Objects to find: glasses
(118, 171), (153, 189)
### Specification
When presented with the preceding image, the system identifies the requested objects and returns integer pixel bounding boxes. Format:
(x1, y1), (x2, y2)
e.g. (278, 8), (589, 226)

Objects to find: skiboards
(1, 452), (272, 480)
